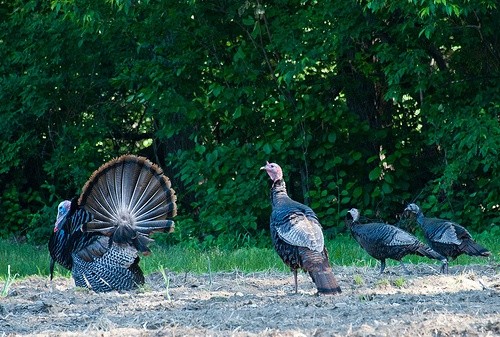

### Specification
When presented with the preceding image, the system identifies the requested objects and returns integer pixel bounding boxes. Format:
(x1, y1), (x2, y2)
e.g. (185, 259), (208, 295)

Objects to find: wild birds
(347, 208), (449, 277)
(47, 153), (178, 293)
(404, 203), (493, 274)
(260, 159), (342, 296)
